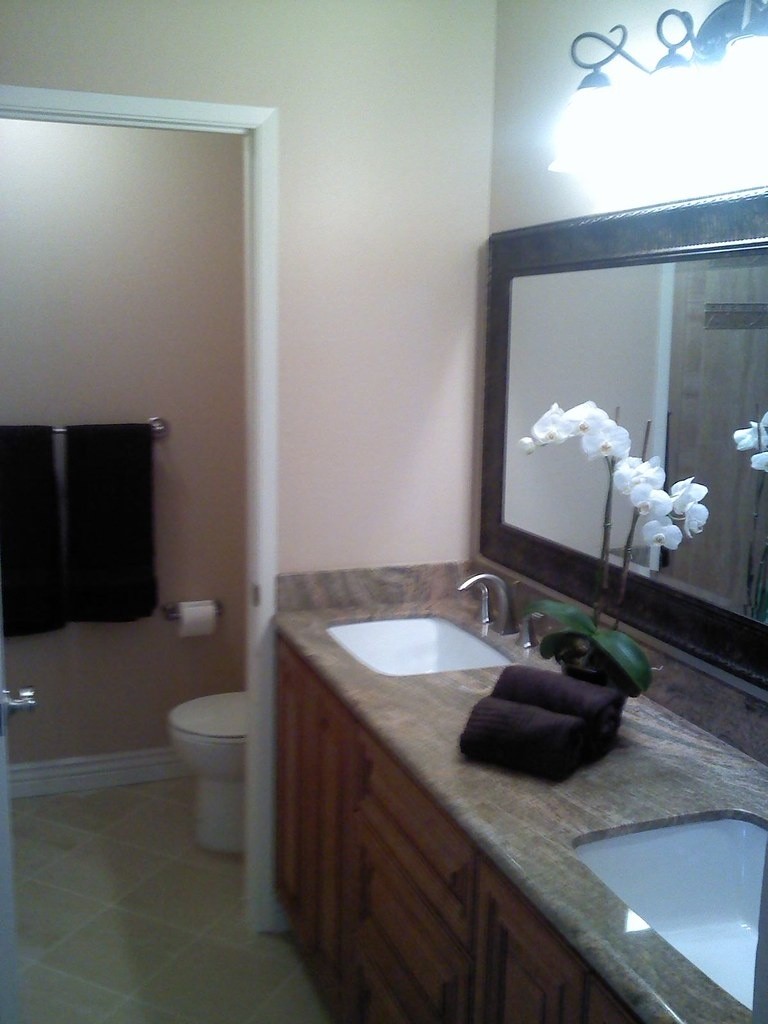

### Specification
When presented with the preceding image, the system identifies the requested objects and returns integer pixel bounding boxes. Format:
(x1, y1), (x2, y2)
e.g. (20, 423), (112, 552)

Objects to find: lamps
(542, 0), (768, 177)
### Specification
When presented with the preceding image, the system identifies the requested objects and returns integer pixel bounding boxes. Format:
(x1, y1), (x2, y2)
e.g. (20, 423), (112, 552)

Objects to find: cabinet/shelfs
(273, 631), (643, 1024)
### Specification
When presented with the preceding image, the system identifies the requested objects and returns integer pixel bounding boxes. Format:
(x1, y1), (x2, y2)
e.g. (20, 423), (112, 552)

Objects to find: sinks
(568, 806), (767, 1012)
(325, 613), (518, 678)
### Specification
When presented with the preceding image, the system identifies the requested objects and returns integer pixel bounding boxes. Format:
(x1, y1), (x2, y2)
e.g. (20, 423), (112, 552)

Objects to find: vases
(560, 648), (628, 711)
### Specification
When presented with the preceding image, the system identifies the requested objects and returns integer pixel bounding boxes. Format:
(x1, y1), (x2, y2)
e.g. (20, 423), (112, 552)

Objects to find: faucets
(456, 571), (521, 636)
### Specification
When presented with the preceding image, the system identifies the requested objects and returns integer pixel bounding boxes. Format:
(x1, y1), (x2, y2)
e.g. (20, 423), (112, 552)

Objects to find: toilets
(164, 690), (247, 854)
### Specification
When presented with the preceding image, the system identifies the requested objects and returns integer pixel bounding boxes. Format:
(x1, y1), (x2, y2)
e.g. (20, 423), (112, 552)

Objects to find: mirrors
(480, 186), (767, 689)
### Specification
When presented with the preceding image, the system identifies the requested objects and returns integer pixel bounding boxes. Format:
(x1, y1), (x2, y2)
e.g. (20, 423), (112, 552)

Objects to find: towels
(458, 661), (626, 784)
(0, 421), (159, 639)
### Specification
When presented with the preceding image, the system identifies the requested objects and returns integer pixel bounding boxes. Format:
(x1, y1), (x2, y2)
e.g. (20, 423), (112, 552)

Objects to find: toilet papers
(176, 599), (218, 637)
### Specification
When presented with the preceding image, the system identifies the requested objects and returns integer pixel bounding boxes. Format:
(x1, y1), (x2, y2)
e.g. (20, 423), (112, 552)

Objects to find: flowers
(733, 404), (768, 625)
(518, 399), (710, 698)
